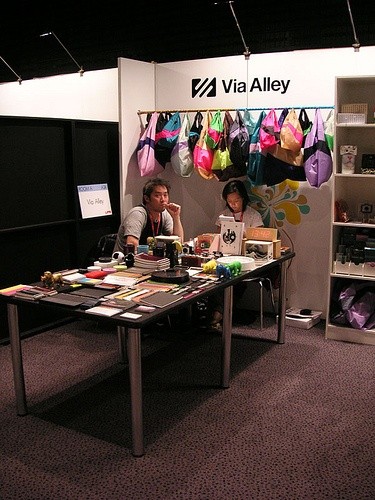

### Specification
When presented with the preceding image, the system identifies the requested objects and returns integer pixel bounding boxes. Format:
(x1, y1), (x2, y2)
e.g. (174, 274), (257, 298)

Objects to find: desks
(0, 252), (295, 457)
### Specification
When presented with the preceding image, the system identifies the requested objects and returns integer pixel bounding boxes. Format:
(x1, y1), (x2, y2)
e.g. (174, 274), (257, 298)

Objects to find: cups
(137, 245), (149, 254)
(123, 245), (134, 256)
(153, 247), (164, 257)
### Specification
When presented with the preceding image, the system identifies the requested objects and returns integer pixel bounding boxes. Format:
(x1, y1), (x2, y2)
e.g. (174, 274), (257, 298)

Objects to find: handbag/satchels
(331, 281), (375, 330)
(136, 107), (333, 188)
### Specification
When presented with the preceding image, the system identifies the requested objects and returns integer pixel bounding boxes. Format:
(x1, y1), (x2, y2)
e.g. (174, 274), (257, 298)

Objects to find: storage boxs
(276, 305), (323, 330)
(337, 102), (368, 125)
(241, 228), (282, 262)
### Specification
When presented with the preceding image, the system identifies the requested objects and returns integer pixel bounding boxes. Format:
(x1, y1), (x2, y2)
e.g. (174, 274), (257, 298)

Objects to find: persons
(207, 179), (266, 331)
(113, 178), (184, 345)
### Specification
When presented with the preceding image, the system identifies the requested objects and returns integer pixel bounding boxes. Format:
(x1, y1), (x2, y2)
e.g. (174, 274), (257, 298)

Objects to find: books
(1, 252), (220, 320)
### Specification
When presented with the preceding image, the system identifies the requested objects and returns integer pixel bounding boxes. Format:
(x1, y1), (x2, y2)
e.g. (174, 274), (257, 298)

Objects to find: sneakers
(210, 321), (223, 332)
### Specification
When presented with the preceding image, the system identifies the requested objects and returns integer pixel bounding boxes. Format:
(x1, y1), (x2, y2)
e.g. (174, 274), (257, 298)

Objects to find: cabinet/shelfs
(324, 76), (375, 346)
